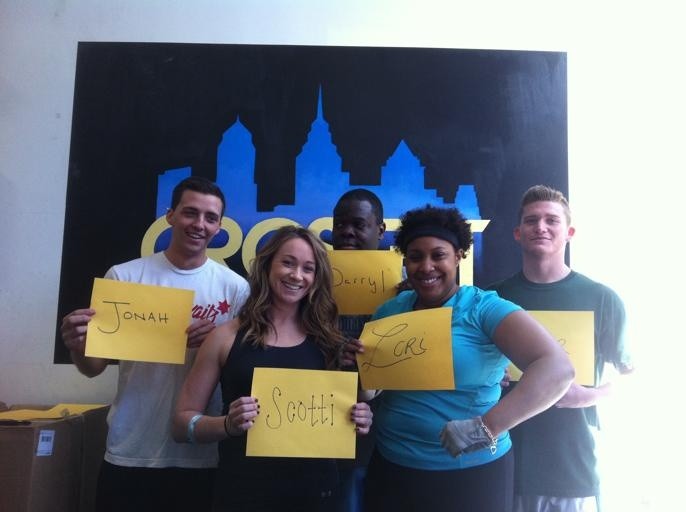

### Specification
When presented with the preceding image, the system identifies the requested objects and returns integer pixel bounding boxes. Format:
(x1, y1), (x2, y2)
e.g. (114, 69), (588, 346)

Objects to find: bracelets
(225, 415), (233, 439)
(482, 424), (497, 456)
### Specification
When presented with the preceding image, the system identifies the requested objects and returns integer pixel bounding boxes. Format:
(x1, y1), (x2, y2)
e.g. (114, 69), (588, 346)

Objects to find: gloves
(440, 416), (492, 458)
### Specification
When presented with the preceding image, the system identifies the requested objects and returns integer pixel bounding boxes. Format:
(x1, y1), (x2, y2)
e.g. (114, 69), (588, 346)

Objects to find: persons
(167, 224), (374, 511)
(329, 188), (408, 511)
(483, 183), (634, 511)
(335, 203), (577, 511)
(59, 176), (254, 511)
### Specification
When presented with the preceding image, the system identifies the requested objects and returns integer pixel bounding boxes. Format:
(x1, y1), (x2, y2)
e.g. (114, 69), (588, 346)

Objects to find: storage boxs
(0, 401), (113, 511)
